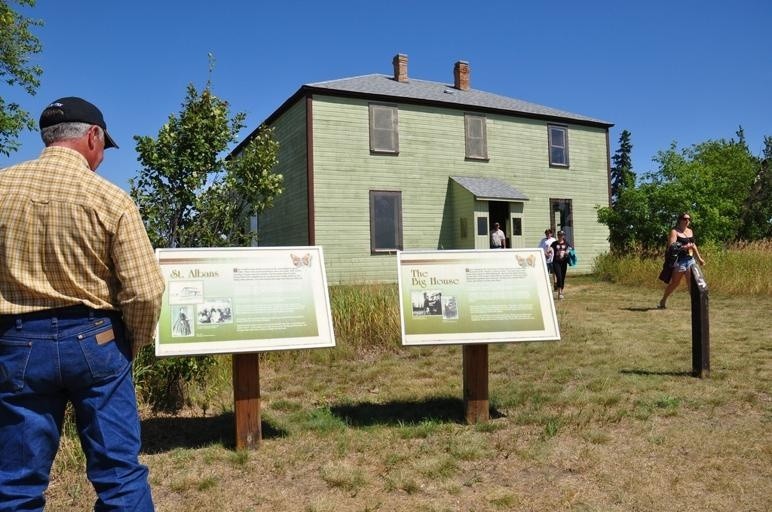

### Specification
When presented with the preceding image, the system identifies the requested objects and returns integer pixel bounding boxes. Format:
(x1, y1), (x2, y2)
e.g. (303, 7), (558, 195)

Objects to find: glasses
(679, 217), (691, 222)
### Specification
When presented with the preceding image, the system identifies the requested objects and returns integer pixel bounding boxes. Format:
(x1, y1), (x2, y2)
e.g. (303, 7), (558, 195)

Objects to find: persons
(490, 222), (506, 249)
(547, 230), (574, 300)
(422, 291), (442, 316)
(656, 212), (705, 310)
(173, 307), (192, 335)
(199, 306), (232, 324)
(1, 97), (165, 512)
(538, 229), (558, 292)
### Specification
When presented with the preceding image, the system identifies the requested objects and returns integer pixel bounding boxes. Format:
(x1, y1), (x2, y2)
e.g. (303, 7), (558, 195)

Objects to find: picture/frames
(395, 247), (561, 347)
(155, 245), (337, 357)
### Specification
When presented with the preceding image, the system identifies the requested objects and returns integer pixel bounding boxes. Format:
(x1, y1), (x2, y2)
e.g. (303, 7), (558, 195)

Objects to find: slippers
(657, 305), (667, 309)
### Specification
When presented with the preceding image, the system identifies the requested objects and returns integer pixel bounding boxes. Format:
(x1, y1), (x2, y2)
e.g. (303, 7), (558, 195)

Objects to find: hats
(39, 96), (119, 150)
(558, 231), (567, 236)
(494, 222), (500, 226)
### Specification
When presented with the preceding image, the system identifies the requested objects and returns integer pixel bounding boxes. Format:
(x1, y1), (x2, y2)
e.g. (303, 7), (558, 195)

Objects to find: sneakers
(558, 293), (564, 300)
